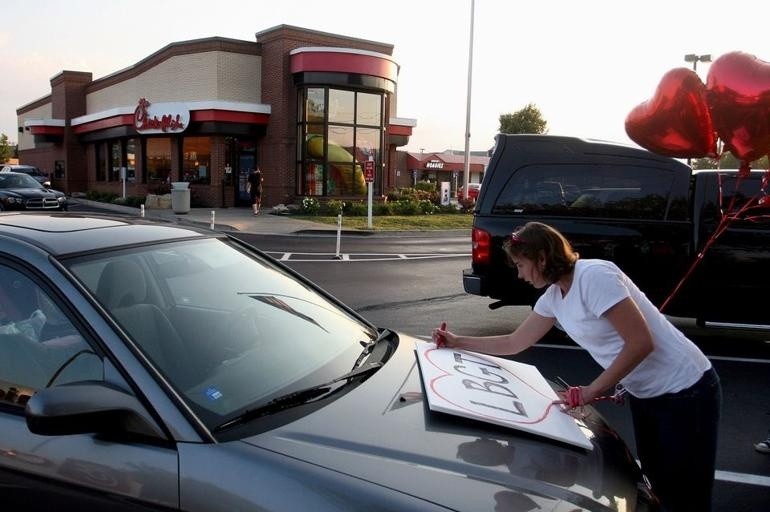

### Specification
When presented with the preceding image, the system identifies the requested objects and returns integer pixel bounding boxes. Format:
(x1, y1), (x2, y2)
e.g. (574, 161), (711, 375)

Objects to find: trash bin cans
(170, 181), (191, 214)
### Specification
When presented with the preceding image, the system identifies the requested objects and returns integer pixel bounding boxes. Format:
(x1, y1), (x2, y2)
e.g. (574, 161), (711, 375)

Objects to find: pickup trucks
(458, 130), (770, 345)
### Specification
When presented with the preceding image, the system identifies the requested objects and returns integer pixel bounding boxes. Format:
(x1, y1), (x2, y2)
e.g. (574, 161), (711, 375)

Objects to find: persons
(431, 222), (723, 512)
(754, 436), (770, 453)
(246, 165), (264, 215)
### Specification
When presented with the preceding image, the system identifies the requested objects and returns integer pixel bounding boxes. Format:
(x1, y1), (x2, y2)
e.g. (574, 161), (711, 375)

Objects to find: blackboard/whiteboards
(415, 340), (594, 451)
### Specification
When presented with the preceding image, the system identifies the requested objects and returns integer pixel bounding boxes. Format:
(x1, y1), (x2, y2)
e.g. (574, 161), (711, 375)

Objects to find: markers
(437, 323), (446, 349)
(556, 376), (569, 390)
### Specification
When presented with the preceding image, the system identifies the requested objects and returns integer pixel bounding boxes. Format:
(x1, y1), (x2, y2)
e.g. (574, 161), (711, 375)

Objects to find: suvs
(0, 170), (71, 212)
(0, 164), (52, 190)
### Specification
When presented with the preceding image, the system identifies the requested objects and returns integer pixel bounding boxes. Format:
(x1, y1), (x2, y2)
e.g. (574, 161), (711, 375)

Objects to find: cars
(112, 164), (136, 181)
(457, 182), (482, 207)
(0, 197), (676, 512)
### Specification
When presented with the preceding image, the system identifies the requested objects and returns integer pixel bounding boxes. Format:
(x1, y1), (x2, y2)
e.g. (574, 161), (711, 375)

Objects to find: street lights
(420, 148), (426, 152)
(681, 51), (713, 168)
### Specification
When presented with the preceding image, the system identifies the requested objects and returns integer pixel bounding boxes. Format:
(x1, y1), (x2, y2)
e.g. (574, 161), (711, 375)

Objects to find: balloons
(625, 68), (720, 160)
(706, 52), (770, 179)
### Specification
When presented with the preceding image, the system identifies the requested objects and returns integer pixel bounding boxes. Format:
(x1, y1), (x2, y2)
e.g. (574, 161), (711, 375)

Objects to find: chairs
(92, 258), (192, 385)
